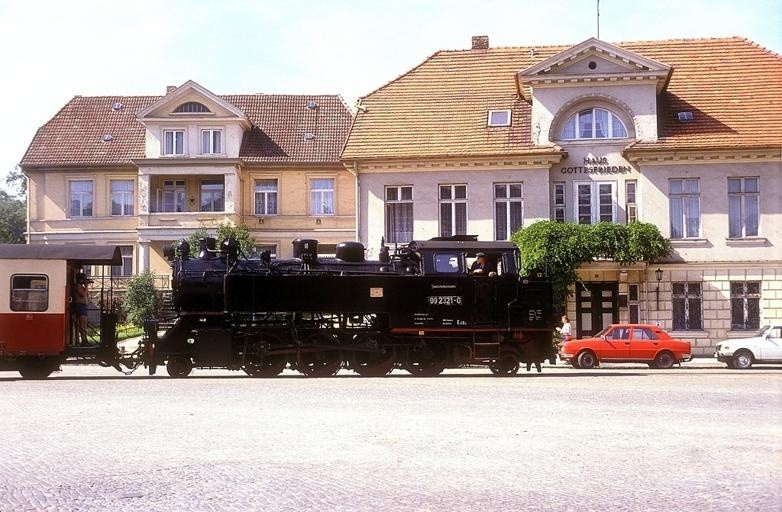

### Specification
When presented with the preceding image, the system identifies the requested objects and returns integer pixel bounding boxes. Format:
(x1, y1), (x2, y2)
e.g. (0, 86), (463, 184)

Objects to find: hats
(476, 252), (488, 258)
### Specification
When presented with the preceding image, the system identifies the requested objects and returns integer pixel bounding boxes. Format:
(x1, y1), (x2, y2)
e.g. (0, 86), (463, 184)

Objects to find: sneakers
(81, 342), (92, 347)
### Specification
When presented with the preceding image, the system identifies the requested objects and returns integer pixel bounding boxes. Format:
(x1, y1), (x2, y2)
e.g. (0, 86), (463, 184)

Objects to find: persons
(75, 271), (94, 347)
(555, 315), (572, 339)
(469, 252), (498, 280)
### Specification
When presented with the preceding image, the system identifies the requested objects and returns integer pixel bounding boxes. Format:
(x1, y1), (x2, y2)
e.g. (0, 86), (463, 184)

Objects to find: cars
(714, 323), (782, 370)
(561, 323), (695, 371)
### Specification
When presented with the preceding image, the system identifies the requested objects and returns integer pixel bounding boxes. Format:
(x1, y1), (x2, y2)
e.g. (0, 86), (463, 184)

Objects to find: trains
(0, 230), (559, 378)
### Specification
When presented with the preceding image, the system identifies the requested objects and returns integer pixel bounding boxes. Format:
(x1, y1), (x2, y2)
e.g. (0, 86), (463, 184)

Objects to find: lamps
(654, 268), (663, 288)
